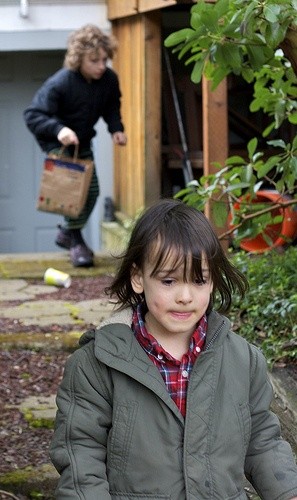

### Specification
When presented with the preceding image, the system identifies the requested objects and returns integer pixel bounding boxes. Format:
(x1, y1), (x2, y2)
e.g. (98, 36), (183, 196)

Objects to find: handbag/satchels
(35, 142), (93, 219)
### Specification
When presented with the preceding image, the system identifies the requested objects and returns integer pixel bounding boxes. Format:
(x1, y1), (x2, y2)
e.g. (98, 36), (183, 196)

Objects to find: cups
(43, 267), (72, 288)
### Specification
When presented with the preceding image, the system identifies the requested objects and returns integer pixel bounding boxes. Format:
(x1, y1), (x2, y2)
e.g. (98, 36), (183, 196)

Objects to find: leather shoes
(69, 243), (95, 268)
(53, 223), (96, 258)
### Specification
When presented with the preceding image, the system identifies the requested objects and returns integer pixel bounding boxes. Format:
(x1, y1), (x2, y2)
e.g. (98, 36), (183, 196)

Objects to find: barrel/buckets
(228, 191), (297, 254)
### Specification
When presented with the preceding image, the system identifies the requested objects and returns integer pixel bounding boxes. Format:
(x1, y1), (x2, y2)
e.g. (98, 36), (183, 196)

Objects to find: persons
(23, 23), (127, 269)
(47, 200), (297, 500)
